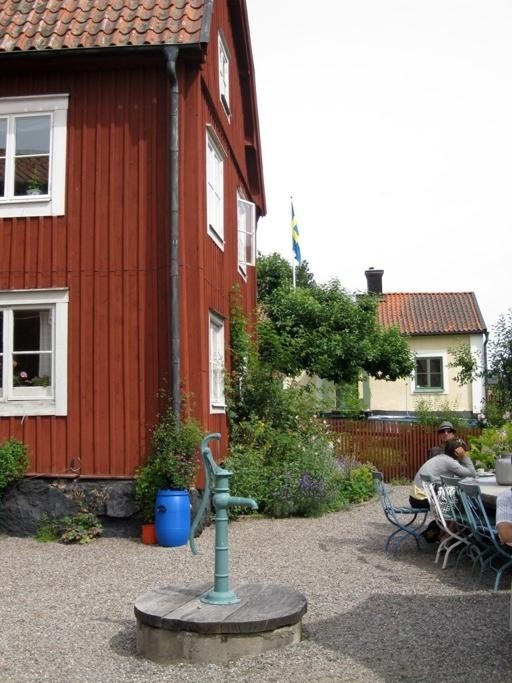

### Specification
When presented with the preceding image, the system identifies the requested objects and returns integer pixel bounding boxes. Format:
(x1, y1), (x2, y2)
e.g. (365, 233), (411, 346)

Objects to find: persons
(427, 421), (456, 460)
(409, 437), (476, 551)
(495, 486), (512, 547)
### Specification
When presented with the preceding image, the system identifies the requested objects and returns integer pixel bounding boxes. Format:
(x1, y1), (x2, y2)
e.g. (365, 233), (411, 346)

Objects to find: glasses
(438, 427), (452, 433)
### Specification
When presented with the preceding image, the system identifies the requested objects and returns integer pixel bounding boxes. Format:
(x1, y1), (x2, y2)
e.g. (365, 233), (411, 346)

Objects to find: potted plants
(25, 168), (43, 195)
(147, 377), (209, 547)
(133, 465), (158, 545)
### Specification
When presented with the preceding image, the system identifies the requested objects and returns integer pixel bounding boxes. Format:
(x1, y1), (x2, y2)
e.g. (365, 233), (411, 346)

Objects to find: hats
(439, 420), (454, 430)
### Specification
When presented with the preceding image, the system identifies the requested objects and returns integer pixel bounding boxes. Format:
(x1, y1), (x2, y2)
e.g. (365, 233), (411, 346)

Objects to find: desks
(456, 471), (512, 504)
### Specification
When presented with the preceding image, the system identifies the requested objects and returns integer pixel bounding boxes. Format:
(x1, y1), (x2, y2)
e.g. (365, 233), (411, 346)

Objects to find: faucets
(213, 490), (259, 510)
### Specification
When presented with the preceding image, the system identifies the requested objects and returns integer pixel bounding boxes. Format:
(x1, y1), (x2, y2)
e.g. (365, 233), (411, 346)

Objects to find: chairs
(437, 476), (489, 576)
(458, 483), (511, 591)
(419, 473), (484, 569)
(371, 470), (429, 557)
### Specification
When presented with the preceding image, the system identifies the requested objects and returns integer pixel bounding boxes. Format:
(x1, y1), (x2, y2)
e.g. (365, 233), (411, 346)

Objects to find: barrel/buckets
(155, 488), (192, 547)
(141, 524), (158, 545)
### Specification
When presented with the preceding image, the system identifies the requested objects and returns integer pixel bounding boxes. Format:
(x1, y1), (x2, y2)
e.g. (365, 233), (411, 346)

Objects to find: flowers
(13, 362), (51, 387)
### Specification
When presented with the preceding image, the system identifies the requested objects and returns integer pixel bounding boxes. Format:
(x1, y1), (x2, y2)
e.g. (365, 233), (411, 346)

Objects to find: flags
(292, 205), (303, 264)
